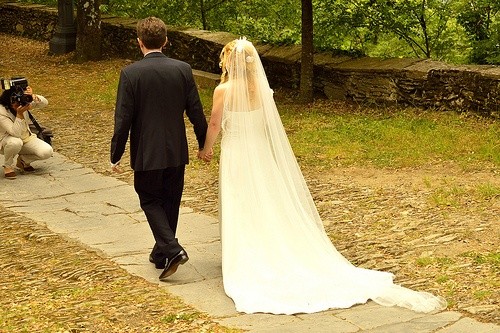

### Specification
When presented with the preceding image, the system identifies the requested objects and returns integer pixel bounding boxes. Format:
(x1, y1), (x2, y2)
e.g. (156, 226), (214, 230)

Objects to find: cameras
(1, 76), (33, 107)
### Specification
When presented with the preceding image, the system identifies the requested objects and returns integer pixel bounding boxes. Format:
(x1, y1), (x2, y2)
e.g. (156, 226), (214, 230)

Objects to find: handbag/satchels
(27, 109), (54, 147)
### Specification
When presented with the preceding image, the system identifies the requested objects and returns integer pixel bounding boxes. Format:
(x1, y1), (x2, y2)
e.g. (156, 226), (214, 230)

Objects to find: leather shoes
(149, 253), (166, 269)
(159, 249), (189, 279)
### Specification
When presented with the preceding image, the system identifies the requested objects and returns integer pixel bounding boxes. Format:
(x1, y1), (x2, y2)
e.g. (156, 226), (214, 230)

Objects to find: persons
(199, 39), (345, 313)
(0, 75), (53, 179)
(110, 17), (214, 279)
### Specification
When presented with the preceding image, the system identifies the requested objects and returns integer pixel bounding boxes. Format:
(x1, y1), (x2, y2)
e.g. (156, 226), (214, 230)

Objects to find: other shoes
(2, 164), (18, 179)
(15, 156), (36, 173)
